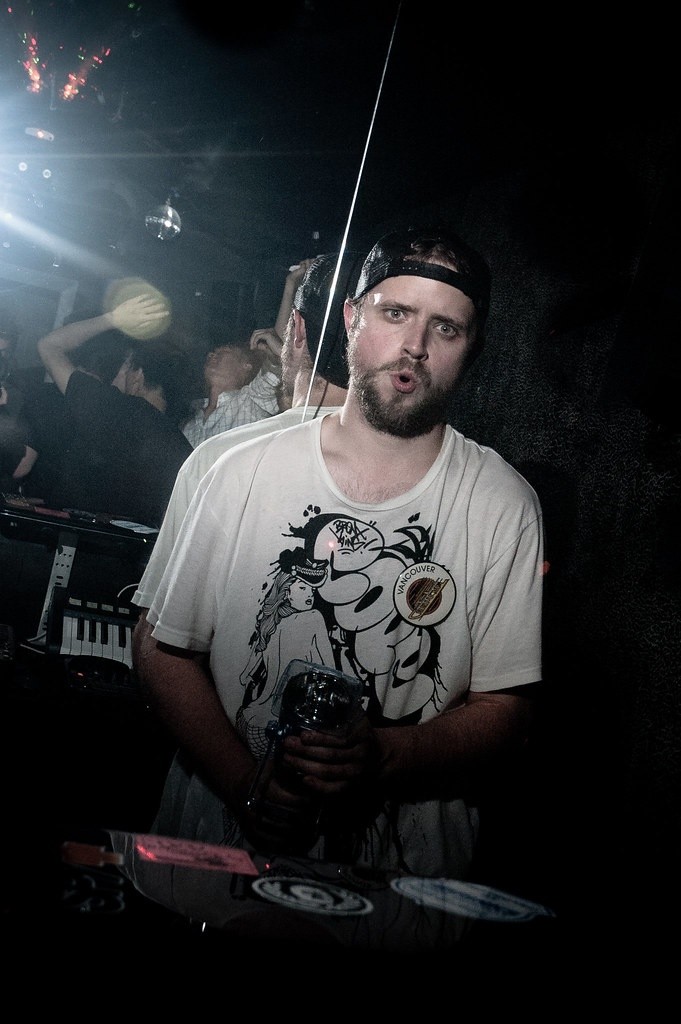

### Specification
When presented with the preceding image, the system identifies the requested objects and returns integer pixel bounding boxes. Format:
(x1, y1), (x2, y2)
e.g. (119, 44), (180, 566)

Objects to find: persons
(0, 249), (377, 535)
(131, 226), (548, 950)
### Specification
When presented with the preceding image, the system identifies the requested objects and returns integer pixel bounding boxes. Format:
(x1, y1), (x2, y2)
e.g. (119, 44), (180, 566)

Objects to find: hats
(352, 226), (491, 328)
(295, 247), (370, 388)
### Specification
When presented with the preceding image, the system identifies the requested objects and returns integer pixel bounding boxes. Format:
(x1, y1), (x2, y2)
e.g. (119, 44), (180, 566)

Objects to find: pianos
(45, 586), (141, 702)
